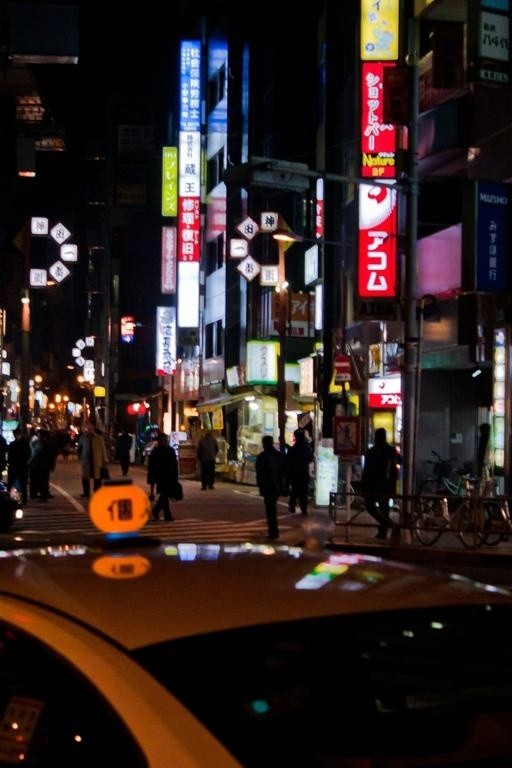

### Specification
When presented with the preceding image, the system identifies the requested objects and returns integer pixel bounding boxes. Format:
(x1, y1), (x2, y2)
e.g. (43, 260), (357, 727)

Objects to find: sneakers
(287, 505), (310, 517)
(375, 521), (401, 541)
(151, 508), (176, 521)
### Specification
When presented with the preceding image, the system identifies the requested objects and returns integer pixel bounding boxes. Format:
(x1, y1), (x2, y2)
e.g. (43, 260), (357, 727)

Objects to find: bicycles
(414, 453), (509, 549)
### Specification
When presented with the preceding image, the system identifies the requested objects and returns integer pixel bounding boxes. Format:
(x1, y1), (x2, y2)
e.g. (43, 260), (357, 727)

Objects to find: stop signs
(334, 355), (351, 373)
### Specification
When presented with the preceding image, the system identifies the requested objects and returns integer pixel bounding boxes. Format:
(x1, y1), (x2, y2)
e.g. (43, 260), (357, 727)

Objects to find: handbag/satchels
(100, 466), (110, 480)
(172, 484), (183, 500)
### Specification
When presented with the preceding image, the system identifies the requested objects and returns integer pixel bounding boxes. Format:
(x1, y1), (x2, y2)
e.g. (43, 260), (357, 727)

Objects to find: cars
(0, 481), (23, 530)
(2, 543), (512, 765)
